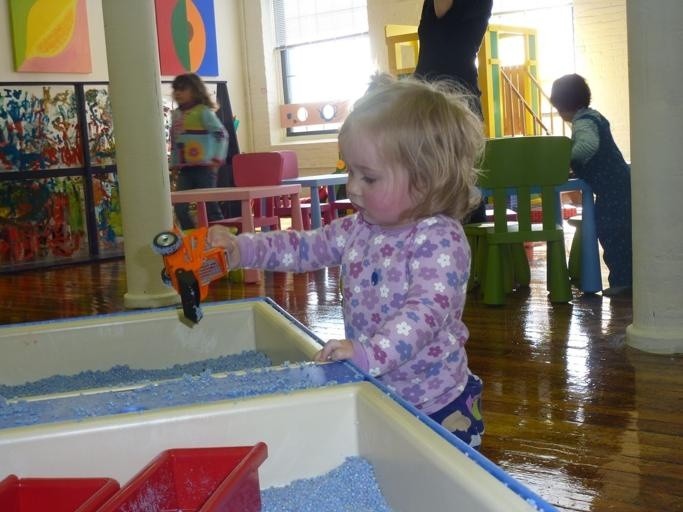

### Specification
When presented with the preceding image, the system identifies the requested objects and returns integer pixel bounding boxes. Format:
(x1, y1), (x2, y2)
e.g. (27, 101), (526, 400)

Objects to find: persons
(208, 74), (490, 451)
(169, 73), (229, 231)
(412, 0), (494, 224)
(551, 74), (631, 297)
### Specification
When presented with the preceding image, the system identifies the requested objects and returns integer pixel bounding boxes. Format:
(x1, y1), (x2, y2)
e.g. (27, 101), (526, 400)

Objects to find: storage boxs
(0, 465), (120, 512)
(94, 437), (269, 512)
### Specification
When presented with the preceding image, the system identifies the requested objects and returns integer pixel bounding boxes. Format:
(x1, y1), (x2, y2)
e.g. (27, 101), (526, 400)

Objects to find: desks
(478, 175), (602, 294)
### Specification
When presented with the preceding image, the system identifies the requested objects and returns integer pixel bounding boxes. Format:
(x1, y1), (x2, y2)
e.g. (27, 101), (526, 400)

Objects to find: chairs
(463, 136), (575, 306)
(172, 151), (353, 285)
